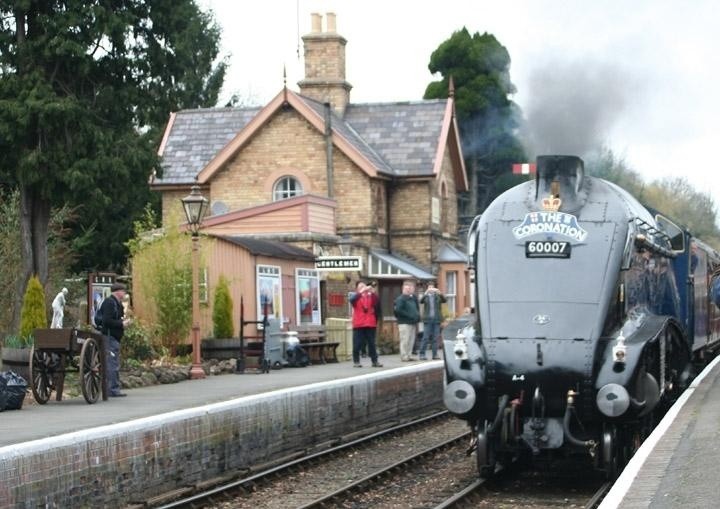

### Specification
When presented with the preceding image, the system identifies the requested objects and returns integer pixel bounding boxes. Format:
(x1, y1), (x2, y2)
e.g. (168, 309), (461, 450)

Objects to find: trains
(440, 154), (720, 485)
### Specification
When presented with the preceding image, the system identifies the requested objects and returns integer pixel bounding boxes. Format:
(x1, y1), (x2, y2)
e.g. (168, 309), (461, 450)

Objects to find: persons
(94, 284), (127, 396)
(392, 282), (417, 361)
(50, 287), (68, 329)
(349, 281), (383, 367)
(420, 281), (446, 360)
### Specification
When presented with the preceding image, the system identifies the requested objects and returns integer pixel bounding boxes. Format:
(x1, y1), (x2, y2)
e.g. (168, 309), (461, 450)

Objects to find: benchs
(288, 324), (340, 365)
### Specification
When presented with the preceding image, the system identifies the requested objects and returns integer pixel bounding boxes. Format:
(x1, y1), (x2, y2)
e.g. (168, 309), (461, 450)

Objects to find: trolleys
(237, 295), (271, 375)
(264, 314), (310, 370)
(28, 326), (109, 404)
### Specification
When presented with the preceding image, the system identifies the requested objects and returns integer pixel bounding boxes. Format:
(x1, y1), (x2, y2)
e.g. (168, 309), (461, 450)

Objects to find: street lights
(180, 172), (208, 378)
